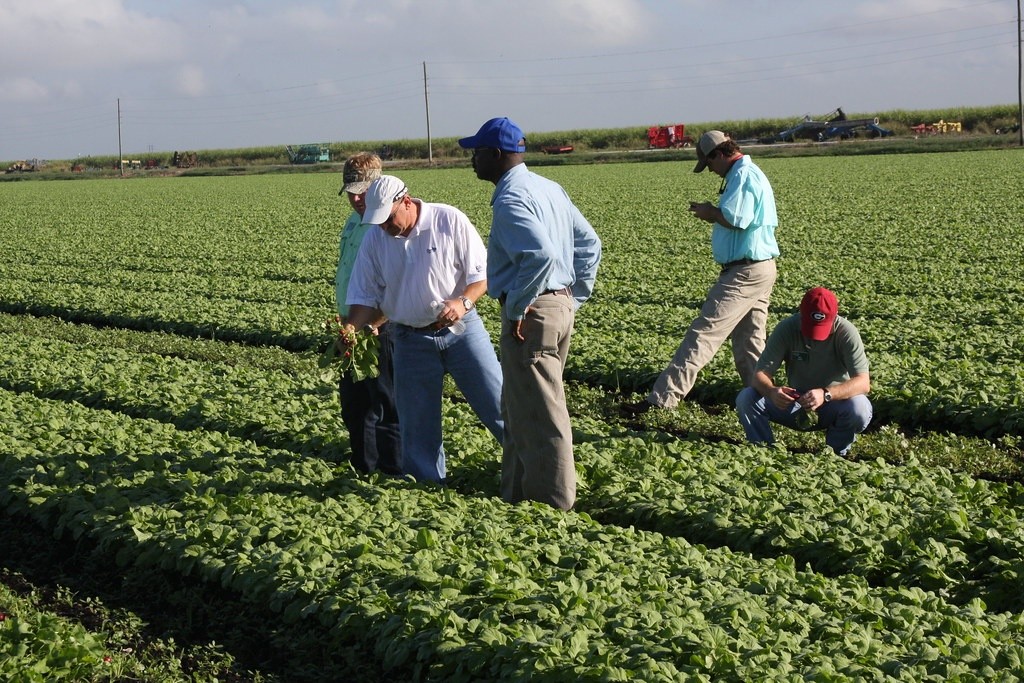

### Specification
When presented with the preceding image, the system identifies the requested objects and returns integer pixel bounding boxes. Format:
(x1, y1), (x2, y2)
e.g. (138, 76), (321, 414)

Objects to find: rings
(449, 318), (454, 322)
(513, 334), (517, 337)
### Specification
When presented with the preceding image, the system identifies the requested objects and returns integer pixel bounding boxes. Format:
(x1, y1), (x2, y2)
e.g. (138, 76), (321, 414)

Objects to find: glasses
(387, 197), (404, 224)
(471, 145), (496, 157)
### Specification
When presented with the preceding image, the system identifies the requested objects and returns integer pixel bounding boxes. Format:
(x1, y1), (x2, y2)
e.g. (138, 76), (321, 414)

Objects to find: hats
(800, 287), (838, 341)
(458, 117), (527, 153)
(693, 130), (729, 173)
(361, 174), (408, 225)
(336, 169), (383, 195)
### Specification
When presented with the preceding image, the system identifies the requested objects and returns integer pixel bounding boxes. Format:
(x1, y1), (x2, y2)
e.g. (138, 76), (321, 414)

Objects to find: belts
(421, 319), (445, 332)
(722, 256), (774, 269)
(498, 287), (573, 307)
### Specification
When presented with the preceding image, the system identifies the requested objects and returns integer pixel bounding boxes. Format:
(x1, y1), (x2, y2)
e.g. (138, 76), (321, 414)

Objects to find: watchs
(361, 324), (375, 333)
(821, 387), (831, 404)
(459, 295), (473, 310)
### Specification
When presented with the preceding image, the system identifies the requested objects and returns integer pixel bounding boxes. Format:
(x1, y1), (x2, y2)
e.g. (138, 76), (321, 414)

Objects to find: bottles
(431, 300), (466, 336)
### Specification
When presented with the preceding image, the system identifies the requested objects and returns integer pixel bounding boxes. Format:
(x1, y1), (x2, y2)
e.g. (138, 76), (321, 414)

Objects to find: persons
(736, 288), (872, 459)
(646, 131), (780, 411)
(334, 153), (400, 476)
(458, 118), (601, 510)
(338, 175), (503, 485)
